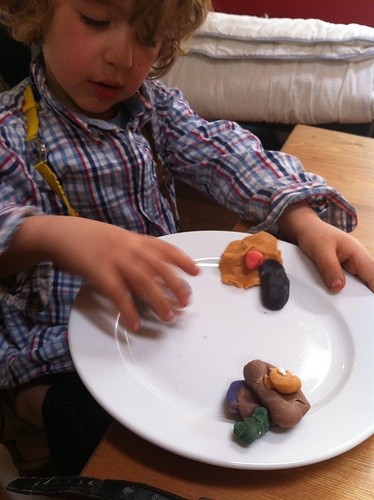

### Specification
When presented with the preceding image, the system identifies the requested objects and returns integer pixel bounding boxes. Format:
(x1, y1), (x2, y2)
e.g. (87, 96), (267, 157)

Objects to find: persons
(0, 0), (374, 472)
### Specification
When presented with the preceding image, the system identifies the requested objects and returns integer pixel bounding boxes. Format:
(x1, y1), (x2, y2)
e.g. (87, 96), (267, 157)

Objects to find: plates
(67, 231), (373, 470)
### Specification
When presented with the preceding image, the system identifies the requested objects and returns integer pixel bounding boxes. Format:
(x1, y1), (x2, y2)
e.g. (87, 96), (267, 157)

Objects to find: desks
(78, 123), (374, 500)
